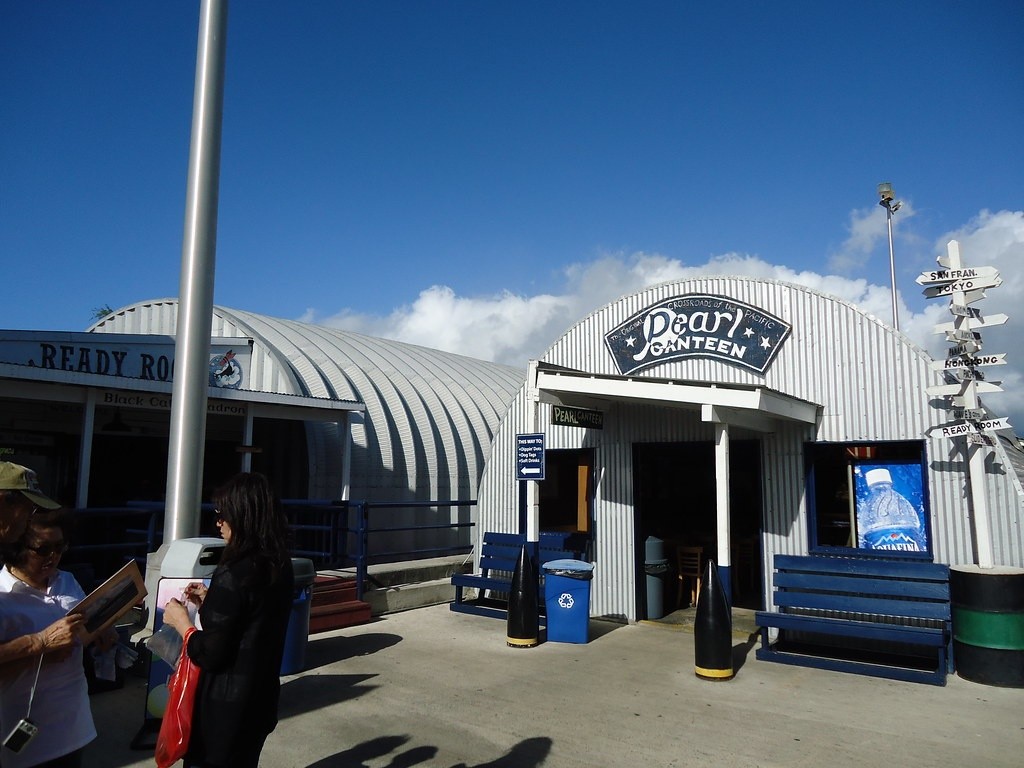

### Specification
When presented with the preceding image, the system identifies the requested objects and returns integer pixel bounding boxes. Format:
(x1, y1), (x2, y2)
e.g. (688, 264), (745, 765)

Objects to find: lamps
(101, 406), (133, 432)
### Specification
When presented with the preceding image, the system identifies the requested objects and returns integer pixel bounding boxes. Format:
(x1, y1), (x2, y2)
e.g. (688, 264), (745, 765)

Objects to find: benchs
(449, 532), (587, 627)
(753, 553), (956, 687)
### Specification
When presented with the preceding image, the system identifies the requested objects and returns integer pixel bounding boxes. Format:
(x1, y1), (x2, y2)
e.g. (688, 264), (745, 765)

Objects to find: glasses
(26, 540), (68, 556)
(213, 509), (224, 523)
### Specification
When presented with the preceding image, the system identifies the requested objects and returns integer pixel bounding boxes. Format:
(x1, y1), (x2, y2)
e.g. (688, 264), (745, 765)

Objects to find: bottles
(857, 468), (923, 552)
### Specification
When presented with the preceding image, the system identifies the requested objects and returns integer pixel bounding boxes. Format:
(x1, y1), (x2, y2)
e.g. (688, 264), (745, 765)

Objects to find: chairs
(672, 532), (753, 608)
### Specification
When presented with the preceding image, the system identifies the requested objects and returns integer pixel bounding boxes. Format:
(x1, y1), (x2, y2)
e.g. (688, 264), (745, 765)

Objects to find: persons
(163, 473), (294, 768)
(0, 459), (120, 768)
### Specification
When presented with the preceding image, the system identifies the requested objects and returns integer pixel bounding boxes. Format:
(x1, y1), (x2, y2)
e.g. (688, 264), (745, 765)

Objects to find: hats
(0, 461), (62, 509)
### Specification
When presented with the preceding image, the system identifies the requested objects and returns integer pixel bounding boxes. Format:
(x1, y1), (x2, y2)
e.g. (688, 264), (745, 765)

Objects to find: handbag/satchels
(153, 628), (201, 768)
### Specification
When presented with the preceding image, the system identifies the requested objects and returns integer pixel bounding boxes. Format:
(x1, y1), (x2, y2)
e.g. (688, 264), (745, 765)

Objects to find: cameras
(3, 718), (38, 755)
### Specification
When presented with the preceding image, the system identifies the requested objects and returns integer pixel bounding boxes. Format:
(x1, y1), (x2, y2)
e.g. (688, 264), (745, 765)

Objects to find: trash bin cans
(279, 557), (316, 674)
(645, 535), (669, 620)
(542, 558), (594, 644)
